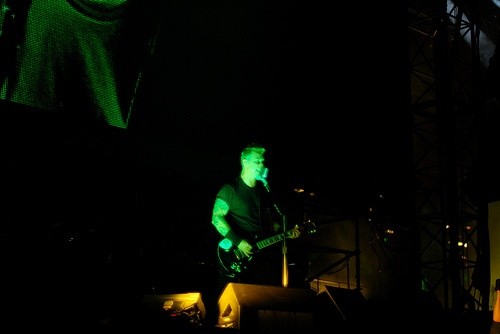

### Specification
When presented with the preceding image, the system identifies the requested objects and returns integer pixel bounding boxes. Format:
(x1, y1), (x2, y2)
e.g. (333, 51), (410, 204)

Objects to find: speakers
(216, 282), (367, 329)
(161, 292), (206, 321)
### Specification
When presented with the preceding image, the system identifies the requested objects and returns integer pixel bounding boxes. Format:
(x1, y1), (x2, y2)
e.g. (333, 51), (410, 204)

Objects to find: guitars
(217, 218), (317, 274)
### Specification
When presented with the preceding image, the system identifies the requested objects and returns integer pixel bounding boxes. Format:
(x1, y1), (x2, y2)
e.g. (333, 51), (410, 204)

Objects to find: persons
(211, 143), (302, 288)
